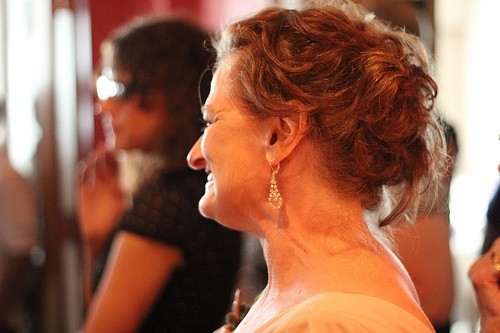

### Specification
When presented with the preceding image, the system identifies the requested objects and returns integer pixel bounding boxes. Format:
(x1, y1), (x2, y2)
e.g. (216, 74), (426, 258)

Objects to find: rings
(492, 253), (499, 274)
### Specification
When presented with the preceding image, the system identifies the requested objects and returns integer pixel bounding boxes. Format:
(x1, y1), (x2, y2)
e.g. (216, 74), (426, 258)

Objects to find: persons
(395, 115), (500, 333)
(74, 17), (244, 333)
(186, 1), (448, 333)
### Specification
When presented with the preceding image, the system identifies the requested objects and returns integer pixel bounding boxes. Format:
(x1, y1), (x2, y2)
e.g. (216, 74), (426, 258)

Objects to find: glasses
(96, 78), (151, 100)
